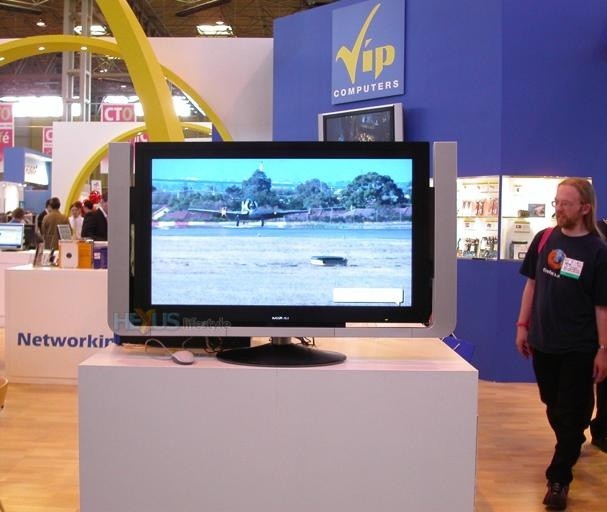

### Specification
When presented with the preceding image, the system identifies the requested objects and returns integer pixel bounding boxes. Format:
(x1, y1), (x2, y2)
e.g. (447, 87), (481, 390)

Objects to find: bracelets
(599, 345), (607, 351)
(515, 320), (530, 328)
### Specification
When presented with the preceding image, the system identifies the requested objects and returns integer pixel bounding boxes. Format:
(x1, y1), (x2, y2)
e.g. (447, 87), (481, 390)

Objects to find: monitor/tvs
(133, 140), (432, 368)
(24, 224), (35, 246)
(56, 224), (72, 240)
(317, 103), (404, 142)
(0, 223), (25, 251)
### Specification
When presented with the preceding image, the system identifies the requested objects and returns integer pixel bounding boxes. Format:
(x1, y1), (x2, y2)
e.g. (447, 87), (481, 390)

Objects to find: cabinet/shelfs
(456, 174), (595, 263)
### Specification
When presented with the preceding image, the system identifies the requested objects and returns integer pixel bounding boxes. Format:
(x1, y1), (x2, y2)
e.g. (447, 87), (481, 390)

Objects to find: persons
(71, 187), (101, 217)
(80, 193), (108, 270)
(38, 198), (75, 250)
(0, 206), (43, 249)
(513, 176), (607, 509)
(585, 218), (607, 452)
(65, 204), (85, 246)
(37, 198), (52, 230)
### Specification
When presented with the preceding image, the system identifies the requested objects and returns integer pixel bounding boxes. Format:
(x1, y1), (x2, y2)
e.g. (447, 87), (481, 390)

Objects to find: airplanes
(188, 198), (309, 228)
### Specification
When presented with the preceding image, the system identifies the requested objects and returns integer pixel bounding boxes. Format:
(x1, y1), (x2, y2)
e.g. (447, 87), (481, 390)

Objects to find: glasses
(551, 200), (583, 209)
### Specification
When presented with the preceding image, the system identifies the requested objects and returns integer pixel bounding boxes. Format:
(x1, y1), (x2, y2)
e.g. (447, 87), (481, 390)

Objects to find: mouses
(171, 350), (196, 364)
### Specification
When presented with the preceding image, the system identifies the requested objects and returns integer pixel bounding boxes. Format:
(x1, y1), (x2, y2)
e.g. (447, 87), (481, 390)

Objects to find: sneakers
(541, 480), (570, 511)
